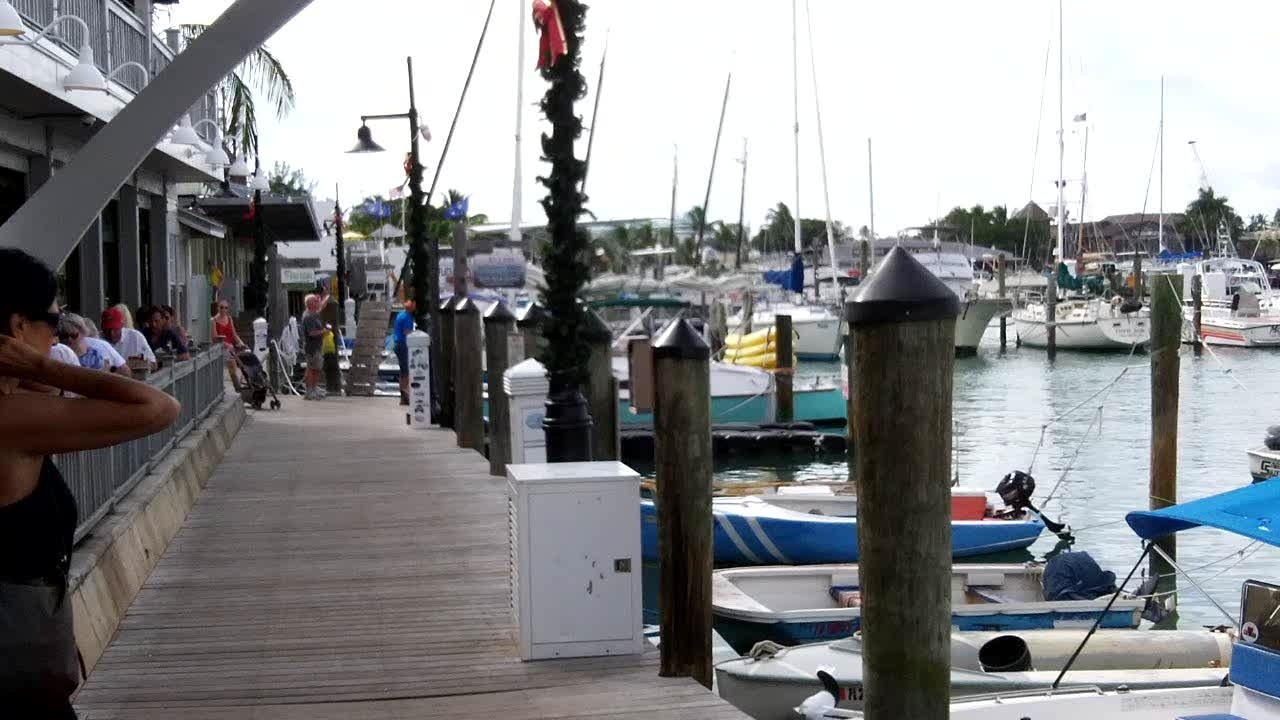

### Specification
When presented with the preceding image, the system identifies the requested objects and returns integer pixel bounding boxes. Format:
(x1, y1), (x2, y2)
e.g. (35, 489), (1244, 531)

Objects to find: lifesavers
(721, 325), (798, 368)
(1230, 278), (1236, 284)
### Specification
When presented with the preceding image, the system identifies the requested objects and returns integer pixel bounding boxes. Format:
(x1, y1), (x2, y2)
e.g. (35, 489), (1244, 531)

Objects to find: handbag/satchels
(1, 578), (88, 698)
(321, 330), (336, 356)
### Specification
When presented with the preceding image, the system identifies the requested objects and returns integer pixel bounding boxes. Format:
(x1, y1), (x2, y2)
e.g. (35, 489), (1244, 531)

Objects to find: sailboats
(440, 1), (1280, 442)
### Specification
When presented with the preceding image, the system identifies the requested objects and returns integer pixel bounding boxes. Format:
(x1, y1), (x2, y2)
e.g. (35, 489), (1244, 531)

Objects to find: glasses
(59, 333), (79, 340)
(219, 306), (229, 310)
(23, 312), (59, 327)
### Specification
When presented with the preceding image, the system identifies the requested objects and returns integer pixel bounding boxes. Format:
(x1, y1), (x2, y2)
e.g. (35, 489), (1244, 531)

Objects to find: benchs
(971, 586), (1024, 606)
(830, 585), (861, 606)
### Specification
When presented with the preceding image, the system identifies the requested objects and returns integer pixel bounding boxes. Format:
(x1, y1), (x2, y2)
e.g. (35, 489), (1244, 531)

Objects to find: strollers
(229, 347), (281, 411)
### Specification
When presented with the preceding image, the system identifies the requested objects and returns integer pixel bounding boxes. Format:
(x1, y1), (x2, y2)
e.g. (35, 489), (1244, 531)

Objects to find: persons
(51, 302), (189, 400)
(210, 301), (248, 396)
(301, 292), (331, 404)
(0, 247), (180, 720)
(209, 261), (224, 312)
(394, 299), (415, 405)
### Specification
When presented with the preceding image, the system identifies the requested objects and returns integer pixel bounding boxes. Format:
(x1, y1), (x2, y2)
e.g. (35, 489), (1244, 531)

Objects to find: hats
(101, 309), (124, 329)
(404, 300), (416, 308)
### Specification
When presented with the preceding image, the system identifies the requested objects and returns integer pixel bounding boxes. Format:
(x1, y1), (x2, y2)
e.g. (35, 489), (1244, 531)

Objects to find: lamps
(218, 135), (252, 176)
(188, 119), (230, 165)
(169, 111), (200, 145)
(0, 15), (108, 93)
(0, 0), (26, 36)
(245, 168), (272, 191)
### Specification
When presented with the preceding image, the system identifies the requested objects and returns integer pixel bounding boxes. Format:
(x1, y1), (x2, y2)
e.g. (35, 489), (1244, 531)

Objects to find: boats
(714, 622), (1239, 720)
(639, 468), (1066, 570)
(1245, 425), (1280, 484)
(710, 554), (1162, 658)
(791, 475), (1280, 719)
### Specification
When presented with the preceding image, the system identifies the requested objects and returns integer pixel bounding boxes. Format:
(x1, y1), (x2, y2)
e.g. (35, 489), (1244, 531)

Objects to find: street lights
(344, 55), (426, 331)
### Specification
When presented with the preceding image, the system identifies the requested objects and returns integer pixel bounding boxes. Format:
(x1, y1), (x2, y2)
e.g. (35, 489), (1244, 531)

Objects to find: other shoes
(314, 389), (326, 398)
(304, 392), (319, 401)
(400, 396), (409, 406)
(235, 385), (244, 393)
